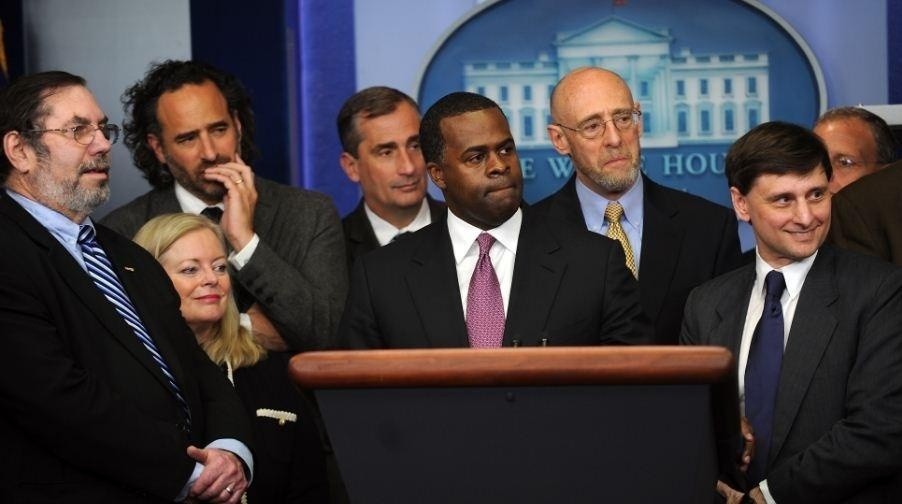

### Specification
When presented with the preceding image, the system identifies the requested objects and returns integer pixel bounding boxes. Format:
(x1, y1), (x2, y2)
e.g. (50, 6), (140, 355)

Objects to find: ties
(467, 232), (506, 349)
(393, 231), (411, 242)
(606, 202), (639, 283)
(744, 272), (786, 489)
(79, 225), (195, 434)
(201, 207), (225, 223)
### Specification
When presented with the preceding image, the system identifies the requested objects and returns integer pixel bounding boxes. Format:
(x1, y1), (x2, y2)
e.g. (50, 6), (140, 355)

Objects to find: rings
(225, 487), (232, 494)
(232, 178), (244, 185)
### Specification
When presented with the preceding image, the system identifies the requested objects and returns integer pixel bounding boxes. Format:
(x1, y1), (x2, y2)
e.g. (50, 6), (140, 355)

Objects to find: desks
(289, 347), (733, 504)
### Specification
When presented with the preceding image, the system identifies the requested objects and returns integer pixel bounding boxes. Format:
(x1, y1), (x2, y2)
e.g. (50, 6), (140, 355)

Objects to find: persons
(338, 92), (647, 350)
(339, 86), (447, 274)
(130, 211), (325, 503)
(683, 119), (901, 504)
(527, 67), (745, 347)
(96, 59), (347, 354)
(0, 71), (256, 503)
(813, 108), (892, 200)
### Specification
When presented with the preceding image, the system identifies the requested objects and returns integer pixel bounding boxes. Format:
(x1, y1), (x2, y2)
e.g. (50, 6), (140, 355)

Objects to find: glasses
(828, 154), (879, 175)
(24, 123), (121, 146)
(552, 110), (642, 141)
